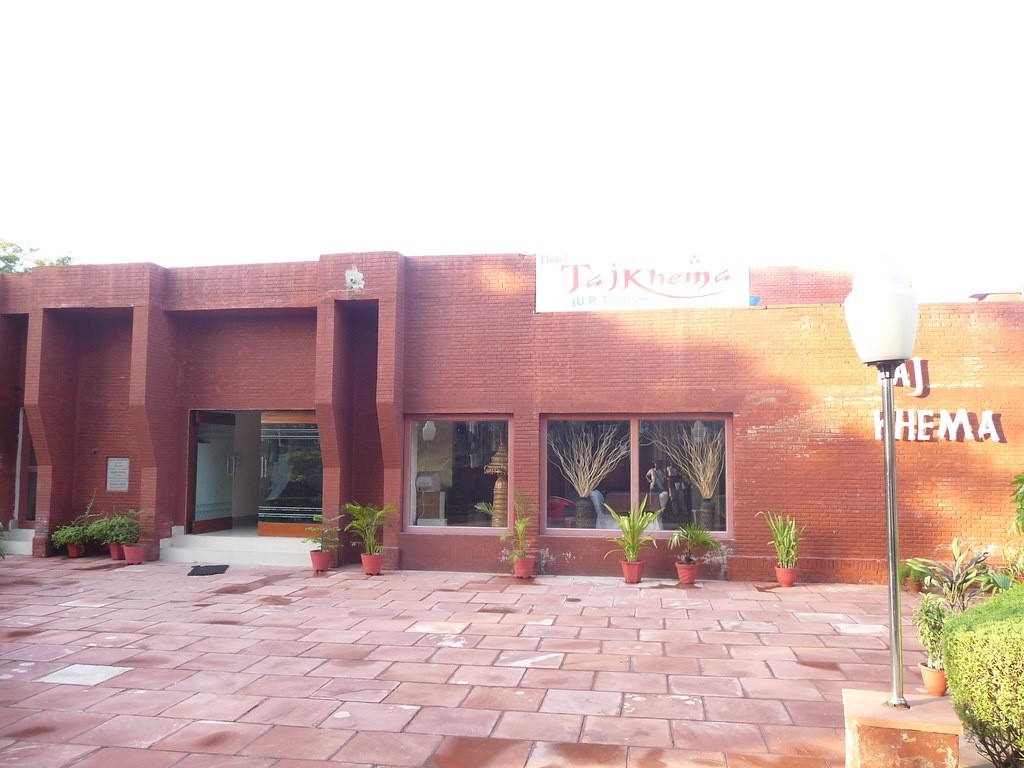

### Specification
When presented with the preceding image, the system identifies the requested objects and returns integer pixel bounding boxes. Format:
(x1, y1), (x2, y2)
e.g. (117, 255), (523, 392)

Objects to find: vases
(575, 497), (595, 528)
(699, 498), (715, 531)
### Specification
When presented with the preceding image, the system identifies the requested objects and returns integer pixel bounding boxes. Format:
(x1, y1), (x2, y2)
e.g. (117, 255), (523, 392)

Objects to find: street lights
(841, 275), (924, 708)
(690, 420), (708, 511)
(421, 419), (436, 472)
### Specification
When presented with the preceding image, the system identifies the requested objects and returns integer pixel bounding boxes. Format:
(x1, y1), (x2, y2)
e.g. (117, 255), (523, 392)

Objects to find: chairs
(547, 496), (577, 528)
(589, 489), (668, 530)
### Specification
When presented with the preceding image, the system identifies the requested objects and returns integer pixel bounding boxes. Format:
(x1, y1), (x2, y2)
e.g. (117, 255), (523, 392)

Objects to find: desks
(605, 492), (660, 513)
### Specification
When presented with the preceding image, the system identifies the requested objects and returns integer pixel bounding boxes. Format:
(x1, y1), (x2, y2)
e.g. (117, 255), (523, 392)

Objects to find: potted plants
(603, 493), (666, 584)
(113, 514), (146, 565)
(898, 563), (929, 593)
(912, 591), (949, 697)
(1012, 559), (1024, 581)
(667, 521), (719, 584)
(300, 514), (346, 572)
(51, 488), (98, 558)
(474, 488), (536, 579)
(340, 499), (397, 576)
(87, 508), (144, 560)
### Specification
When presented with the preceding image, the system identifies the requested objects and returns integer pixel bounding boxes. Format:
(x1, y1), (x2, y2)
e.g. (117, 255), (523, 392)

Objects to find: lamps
(843, 285), (921, 708)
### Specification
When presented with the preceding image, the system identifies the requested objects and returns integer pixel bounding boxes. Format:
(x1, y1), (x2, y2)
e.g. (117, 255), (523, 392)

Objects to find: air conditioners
(754, 503), (808, 587)
